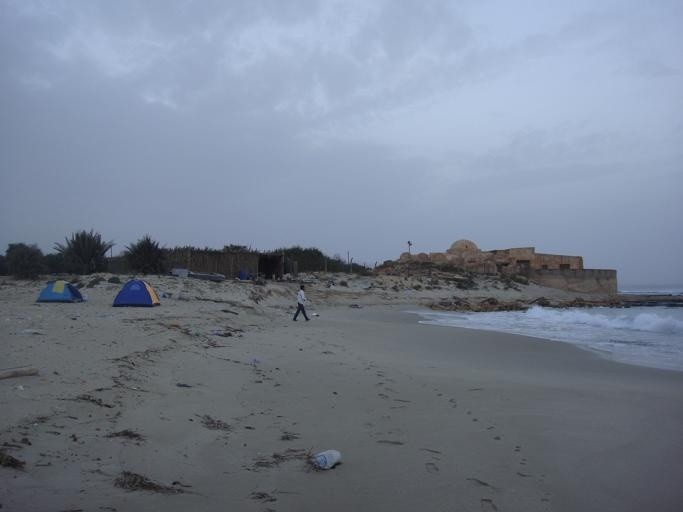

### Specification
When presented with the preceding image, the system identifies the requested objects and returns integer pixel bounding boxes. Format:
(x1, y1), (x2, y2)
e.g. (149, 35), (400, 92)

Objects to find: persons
(292, 285), (311, 321)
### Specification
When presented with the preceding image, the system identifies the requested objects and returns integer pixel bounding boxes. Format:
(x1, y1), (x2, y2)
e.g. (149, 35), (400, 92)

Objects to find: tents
(36, 280), (84, 303)
(111, 279), (160, 307)
